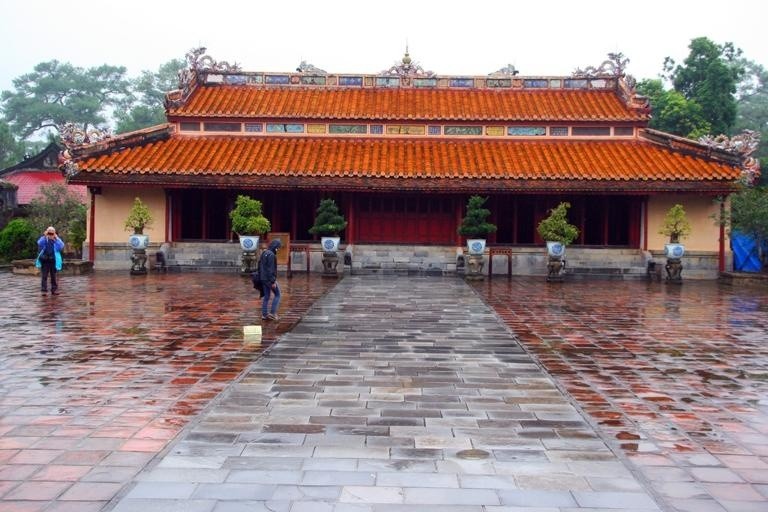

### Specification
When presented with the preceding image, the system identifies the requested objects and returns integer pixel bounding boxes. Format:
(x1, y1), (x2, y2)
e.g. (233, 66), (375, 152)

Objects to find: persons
(35, 226), (65, 296)
(258, 240), (281, 320)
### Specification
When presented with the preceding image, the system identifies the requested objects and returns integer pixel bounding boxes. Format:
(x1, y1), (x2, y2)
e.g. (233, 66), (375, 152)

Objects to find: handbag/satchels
(40, 255), (49, 262)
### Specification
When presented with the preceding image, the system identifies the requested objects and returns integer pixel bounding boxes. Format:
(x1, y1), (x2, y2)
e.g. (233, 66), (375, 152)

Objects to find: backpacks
(252, 272), (260, 290)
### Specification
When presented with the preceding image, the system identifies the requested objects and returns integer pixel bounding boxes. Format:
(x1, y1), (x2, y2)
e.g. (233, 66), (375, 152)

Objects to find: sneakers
(42, 292), (48, 296)
(51, 290), (60, 295)
(262, 313), (281, 320)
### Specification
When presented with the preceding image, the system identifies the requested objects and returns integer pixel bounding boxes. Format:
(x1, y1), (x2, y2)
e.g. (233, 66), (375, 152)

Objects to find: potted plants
(307, 197), (349, 252)
(123, 196), (154, 249)
(656, 203), (693, 259)
(229, 193), (274, 252)
(536, 200), (581, 259)
(455, 194), (499, 254)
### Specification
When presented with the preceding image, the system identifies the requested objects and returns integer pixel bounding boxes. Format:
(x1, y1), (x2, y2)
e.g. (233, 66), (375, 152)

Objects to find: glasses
(49, 232), (55, 236)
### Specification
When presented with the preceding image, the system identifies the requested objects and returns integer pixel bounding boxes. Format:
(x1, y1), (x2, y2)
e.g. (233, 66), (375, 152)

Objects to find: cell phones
(48, 232), (54, 236)
